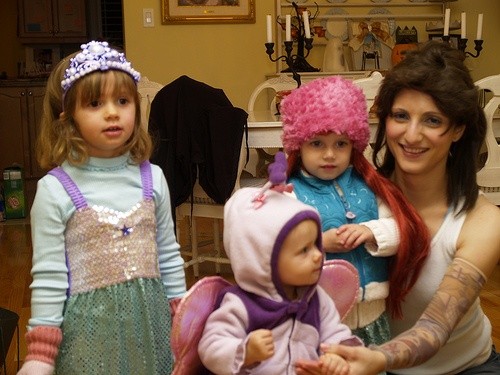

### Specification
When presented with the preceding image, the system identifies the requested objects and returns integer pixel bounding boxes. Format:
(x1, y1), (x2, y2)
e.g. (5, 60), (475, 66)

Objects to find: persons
(196, 187), (365, 375)
(263, 73), (430, 375)
(292, 41), (500, 375)
(15, 40), (188, 375)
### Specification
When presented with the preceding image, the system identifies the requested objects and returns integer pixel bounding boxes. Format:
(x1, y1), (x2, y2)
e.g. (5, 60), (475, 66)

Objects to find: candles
(286, 15), (291, 40)
(443, 9), (450, 35)
(303, 11), (311, 38)
(461, 12), (466, 38)
(267, 15), (272, 43)
(477, 13), (483, 39)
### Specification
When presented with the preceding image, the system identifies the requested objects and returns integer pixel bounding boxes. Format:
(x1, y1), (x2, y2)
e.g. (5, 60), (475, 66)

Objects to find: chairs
(472, 74), (500, 208)
(248, 74), (299, 178)
(354, 70), (386, 114)
(135, 75), (271, 278)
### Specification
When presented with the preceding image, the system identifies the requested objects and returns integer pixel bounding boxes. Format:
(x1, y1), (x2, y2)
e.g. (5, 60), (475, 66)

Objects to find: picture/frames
(161, 0), (256, 24)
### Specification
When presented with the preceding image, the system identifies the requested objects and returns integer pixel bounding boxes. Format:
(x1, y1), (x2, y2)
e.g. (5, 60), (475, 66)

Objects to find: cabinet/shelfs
(0, 0), (87, 180)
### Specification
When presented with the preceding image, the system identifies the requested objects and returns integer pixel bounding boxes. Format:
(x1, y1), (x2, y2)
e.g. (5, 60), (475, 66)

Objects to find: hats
(279, 76), (370, 157)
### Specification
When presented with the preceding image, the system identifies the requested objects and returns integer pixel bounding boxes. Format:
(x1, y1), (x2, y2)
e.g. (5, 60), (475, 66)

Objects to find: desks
(244, 110), (385, 171)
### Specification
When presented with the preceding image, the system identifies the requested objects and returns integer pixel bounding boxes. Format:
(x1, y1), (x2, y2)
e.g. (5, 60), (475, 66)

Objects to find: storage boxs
(2, 168), (26, 220)
